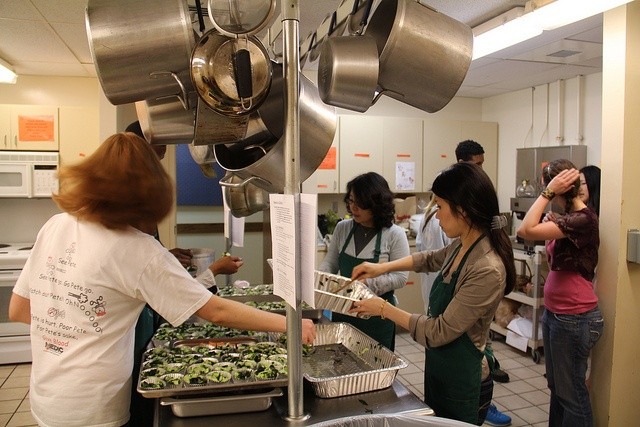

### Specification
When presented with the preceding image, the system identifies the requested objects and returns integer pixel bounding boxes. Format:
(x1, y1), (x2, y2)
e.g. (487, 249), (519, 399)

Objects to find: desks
(154, 287), (434, 425)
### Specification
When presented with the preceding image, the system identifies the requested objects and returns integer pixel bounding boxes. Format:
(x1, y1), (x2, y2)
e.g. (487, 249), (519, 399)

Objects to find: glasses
(345, 199), (360, 206)
(580, 182), (586, 185)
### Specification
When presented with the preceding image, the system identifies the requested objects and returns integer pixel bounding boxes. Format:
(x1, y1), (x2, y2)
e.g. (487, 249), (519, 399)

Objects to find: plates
(313, 270), (378, 320)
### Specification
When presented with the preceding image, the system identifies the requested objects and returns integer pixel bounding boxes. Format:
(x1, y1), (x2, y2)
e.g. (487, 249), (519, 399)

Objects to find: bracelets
(381, 300), (387, 321)
(540, 187), (555, 199)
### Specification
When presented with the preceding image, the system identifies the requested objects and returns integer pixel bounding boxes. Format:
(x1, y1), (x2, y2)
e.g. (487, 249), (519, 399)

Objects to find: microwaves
(0, 150), (59, 198)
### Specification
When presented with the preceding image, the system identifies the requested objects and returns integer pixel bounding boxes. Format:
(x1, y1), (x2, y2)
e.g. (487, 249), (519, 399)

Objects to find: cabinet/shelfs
(0, 105), (58, 152)
(313, 194), (437, 330)
(515, 145), (587, 198)
(303, 117), (340, 194)
(340, 114), (425, 194)
(424, 119), (499, 193)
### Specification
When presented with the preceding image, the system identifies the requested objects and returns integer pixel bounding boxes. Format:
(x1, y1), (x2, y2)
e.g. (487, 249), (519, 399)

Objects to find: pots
(135, 96), (195, 145)
(84, 0), (195, 111)
(363, 1), (473, 113)
(317, 34), (379, 113)
(225, 171), (271, 217)
(188, 140), (216, 164)
(213, 63), (337, 194)
(194, 96), (250, 147)
(189, 25), (273, 118)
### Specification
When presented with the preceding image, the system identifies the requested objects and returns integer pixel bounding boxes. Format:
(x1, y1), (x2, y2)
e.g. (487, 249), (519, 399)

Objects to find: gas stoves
(0, 243), (36, 270)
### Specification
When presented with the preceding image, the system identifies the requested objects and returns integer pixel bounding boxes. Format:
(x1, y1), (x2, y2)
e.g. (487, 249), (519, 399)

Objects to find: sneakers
(492, 370), (509, 384)
(483, 404), (512, 427)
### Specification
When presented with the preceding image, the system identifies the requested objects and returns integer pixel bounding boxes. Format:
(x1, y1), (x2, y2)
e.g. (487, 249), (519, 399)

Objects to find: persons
(193, 254), (245, 300)
(515, 157), (605, 427)
(7, 132), (318, 427)
(414, 189), (458, 315)
(455, 139), (511, 384)
(351, 161), (514, 424)
(124, 121), (193, 383)
(576, 165), (600, 214)
(320, 171), (411, 354)
